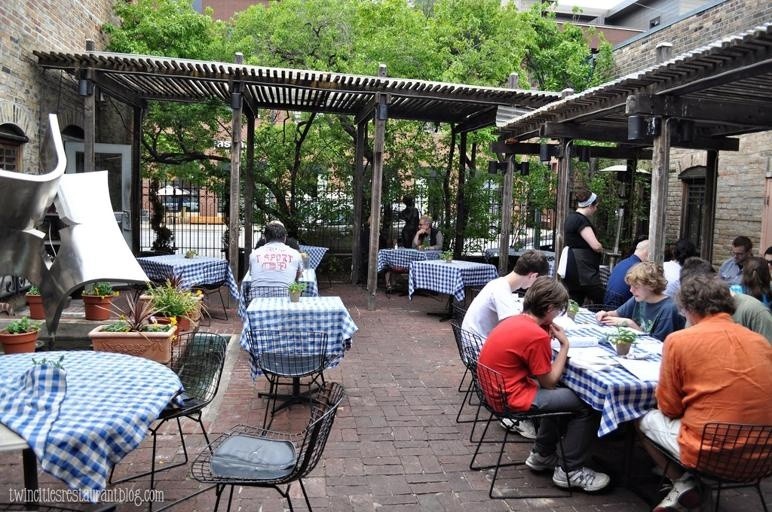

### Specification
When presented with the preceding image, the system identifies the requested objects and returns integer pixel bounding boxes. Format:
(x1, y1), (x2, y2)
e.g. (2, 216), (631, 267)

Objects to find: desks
(0, 350), (184, 512)
(295, 245), (329, 285)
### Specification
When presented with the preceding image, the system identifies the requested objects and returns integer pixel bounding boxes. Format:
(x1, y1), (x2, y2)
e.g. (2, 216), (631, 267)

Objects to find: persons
(254, 219), (300, 250)
(462, 186), (772, 511)
(246, 222), (303, 304)
(398, 193), (421, 248)
(360, 216), (396, 290)
(412, 215), (444, 251)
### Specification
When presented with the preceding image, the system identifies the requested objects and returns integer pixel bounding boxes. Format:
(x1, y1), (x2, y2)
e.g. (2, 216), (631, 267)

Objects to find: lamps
(539, 141), (559, 161)
(233, 87), (242, 109)
(571, 144), (591, 162)
(78, 76), (92, 97)
(488, 160), (499, 174)
(376, 101), (388, 120)
(516, 160), (529, 176)
(626, 114), (647, 141)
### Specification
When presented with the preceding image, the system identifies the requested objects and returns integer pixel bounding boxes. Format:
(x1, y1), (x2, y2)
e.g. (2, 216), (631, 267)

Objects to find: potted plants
(81, 279), (119, 321)
(24, 282), (46, 319)
(86, 290), (178, 365)
(1, 315), (43, 354)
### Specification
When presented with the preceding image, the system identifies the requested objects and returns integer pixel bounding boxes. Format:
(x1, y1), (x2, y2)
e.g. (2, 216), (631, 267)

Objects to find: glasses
(419, 224), (428, 226)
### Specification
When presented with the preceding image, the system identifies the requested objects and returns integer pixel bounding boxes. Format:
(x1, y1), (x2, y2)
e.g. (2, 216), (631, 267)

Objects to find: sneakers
(554, 466), (610, 491)
(653, 472), (702, 512)
(526, 451), (559, 471)
(501, 418), (537, 439)
